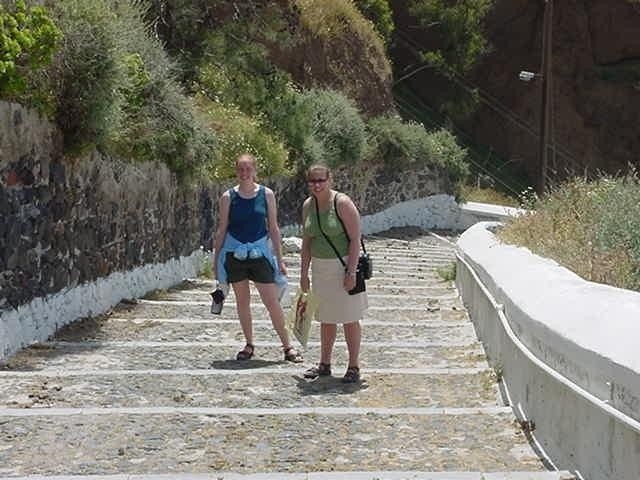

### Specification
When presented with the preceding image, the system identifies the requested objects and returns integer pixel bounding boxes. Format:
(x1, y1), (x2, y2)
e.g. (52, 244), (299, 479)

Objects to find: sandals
(237, 343), (254, 360)
(284, 347), (305, 363)
(342, 366), (360, 383)
(303, 362), (331, 378)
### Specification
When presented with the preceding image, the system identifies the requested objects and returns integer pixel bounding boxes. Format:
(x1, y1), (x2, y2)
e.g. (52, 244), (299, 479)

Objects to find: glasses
(307, 177), (329, 187)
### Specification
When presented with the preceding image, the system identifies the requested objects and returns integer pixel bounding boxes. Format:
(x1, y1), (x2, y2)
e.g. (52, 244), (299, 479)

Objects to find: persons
(299, 163), (367, 383)
(211, 152), (306, 365)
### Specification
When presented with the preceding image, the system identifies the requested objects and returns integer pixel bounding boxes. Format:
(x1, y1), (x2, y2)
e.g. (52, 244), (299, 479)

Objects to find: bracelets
(345, 269), (357, 278)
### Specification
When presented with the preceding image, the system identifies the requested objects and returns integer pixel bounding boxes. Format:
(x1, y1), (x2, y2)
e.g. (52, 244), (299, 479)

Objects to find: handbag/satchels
(358, 253), (373, 279)
(287, 288), (314, 347)
(345, 267), (365, 295)
